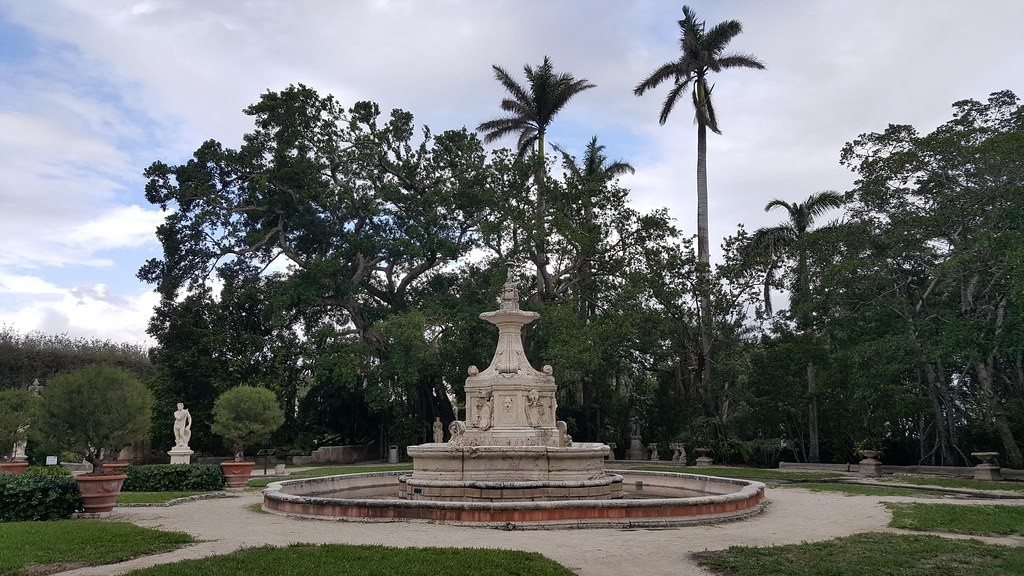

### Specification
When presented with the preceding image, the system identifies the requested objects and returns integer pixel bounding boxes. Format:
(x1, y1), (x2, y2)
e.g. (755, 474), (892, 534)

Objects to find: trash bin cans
(388, 445), (399, 464)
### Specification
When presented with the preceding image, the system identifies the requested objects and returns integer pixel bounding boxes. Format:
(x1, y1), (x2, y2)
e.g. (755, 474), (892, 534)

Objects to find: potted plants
(209, 380), (285, 488)
(689, 437), (716, 457)
(0, 361), (156, 513)
(961, 421), (1003, 464)
(852, 437), (886, 459)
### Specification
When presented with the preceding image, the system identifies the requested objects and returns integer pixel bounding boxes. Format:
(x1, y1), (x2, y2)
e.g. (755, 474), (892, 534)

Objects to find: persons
(433, 417), (443, 443)
(28, 378), (39, 396)
(13, 418), (30, 455)
(173, 403), (192, 447)
(629, 416), (641, 438)
